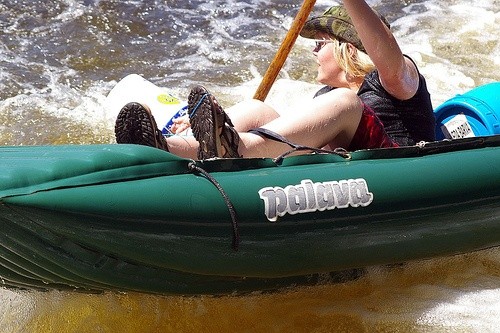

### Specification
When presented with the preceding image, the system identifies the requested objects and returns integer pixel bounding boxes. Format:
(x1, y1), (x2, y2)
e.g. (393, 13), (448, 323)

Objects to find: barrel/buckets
(102, 73), (189, 138)
(428, 74), (500, 138)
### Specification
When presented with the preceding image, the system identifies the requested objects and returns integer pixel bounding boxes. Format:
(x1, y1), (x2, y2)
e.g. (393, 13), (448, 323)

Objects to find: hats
(299, 5), (390, 55)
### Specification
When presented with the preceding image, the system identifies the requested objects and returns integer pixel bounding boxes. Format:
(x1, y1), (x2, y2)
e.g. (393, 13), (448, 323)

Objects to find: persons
(112, 0), (439, 159)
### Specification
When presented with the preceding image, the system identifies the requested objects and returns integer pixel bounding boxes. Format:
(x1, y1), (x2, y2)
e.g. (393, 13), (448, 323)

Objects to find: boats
(0, 81), (499, 295)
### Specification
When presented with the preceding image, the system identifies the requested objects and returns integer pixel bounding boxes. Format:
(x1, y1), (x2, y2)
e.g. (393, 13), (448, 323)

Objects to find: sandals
(188, 85), (242, 159)
(114, 102), (169, 152)
